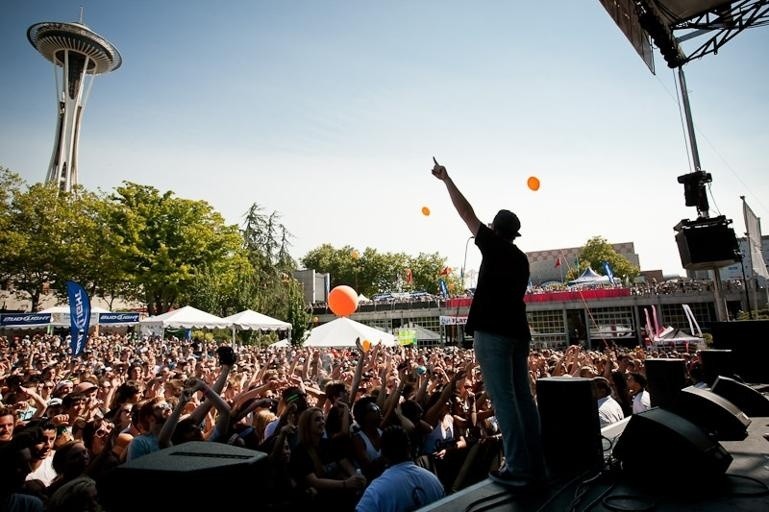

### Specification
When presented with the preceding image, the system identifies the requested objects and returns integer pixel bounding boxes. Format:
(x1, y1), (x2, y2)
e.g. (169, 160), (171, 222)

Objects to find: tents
(269, 318), (398, 348)
(139, 305), (230, 329)
(223, 309), (290, 331)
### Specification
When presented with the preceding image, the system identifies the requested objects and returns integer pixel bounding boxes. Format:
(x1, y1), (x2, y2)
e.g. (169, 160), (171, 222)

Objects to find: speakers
(535, 376), (604, 478)
(608, 405), (733, 500)
(111, 439), (270, 511)
(644, 357), (686, 411)
(711, 319), (769, 384)
(676, 384), (752, 441)
(700, 349), (733, 386)
(675, 228), (740, 271)
(711, 375), (769, 418)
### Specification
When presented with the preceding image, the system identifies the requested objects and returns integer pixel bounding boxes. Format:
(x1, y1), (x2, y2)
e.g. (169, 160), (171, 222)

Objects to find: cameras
(218, 346), (236, 365)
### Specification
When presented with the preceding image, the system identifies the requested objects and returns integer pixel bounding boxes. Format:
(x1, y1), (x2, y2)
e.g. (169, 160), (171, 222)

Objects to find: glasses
(92, 422), (115, 438)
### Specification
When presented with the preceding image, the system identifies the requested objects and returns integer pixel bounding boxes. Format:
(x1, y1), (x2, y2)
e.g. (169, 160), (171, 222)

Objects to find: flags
(69, 281), (90, 356)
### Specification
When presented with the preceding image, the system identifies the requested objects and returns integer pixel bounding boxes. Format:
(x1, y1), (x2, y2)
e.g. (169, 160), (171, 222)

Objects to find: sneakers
(488, 470), (527, 486)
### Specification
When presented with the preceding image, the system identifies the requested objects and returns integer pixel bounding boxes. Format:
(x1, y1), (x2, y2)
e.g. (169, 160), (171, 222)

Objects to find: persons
(0, 324), (701, 511)
(631, 276), (741, 296)
(431, 157), (540, 487)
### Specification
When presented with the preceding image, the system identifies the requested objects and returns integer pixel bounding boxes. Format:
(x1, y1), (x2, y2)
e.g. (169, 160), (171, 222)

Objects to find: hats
(46, 380), (99, 409)
(488, 210), (521, 236)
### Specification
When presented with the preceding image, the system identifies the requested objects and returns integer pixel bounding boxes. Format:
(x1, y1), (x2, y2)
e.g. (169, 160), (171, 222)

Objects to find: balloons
(328, 284), (360, 318)
(422, 207), (429, 217)
(528, 176), (539, 191)
